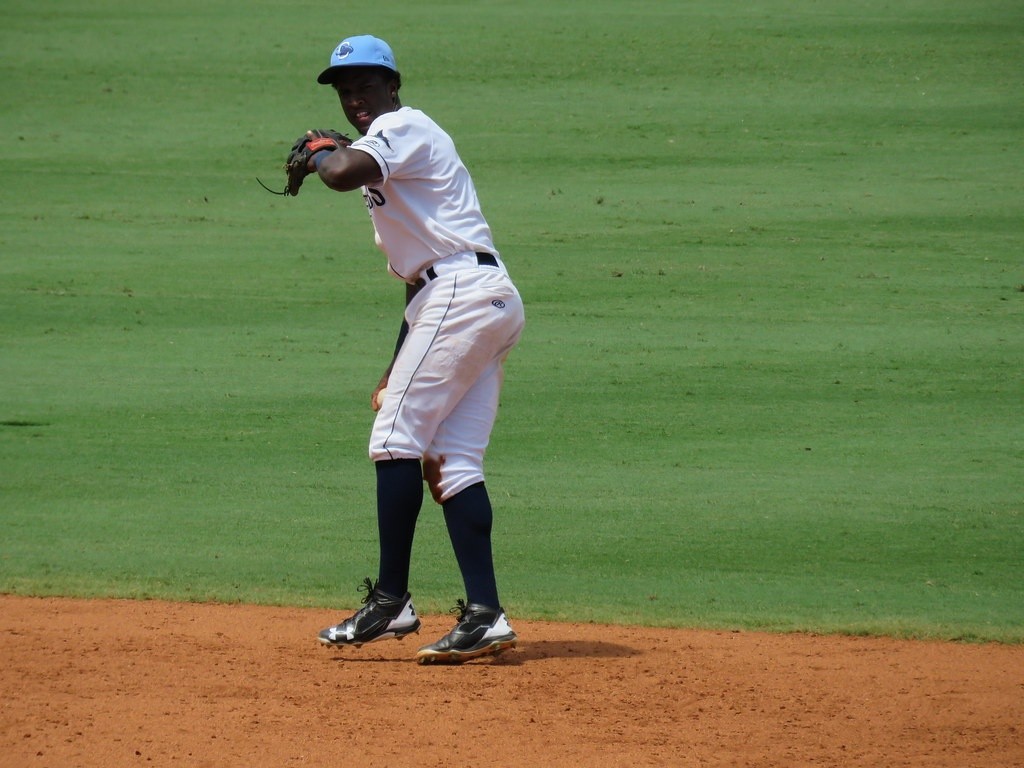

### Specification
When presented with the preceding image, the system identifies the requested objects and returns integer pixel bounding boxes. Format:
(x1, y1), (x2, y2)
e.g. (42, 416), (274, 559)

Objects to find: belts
(415, 252), (499, 293)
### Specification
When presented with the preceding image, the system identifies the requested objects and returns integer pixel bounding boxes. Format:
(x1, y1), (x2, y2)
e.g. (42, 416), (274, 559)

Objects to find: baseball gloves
(285, 127), (357, 194)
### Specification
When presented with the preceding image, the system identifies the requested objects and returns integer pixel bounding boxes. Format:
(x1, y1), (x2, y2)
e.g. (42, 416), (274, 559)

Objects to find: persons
(285, 35), (526, 665)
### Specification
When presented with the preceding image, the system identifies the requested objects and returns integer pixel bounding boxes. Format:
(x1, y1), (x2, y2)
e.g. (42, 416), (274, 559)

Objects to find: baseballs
(375, 387), (389, 410)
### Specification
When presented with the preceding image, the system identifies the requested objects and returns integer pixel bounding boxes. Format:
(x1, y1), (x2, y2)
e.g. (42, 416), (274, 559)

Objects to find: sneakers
(317, 578), (421, 650)
(415, 600), (517, 665)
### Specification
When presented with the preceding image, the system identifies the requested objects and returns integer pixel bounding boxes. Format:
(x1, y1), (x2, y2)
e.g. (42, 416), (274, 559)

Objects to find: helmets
(317, 36), (400, 84)
